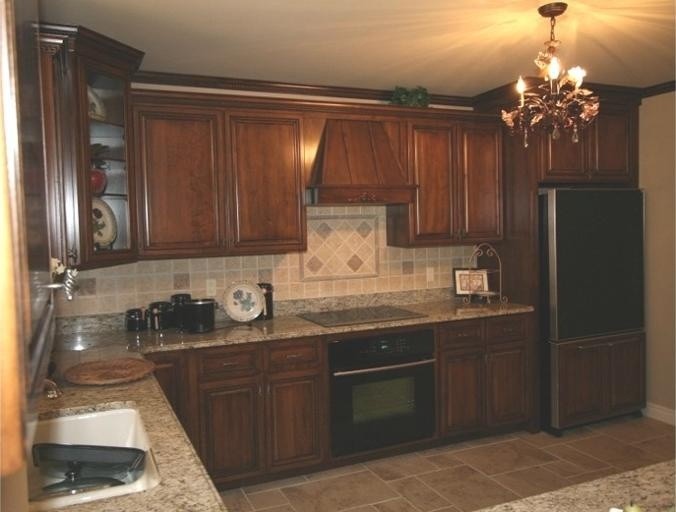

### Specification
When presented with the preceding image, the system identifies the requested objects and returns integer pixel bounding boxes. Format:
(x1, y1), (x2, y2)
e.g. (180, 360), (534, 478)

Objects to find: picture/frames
(453, 267), (490, 296)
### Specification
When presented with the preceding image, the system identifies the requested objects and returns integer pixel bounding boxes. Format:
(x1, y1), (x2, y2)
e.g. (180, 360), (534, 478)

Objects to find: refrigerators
(537, 189), (648, 431)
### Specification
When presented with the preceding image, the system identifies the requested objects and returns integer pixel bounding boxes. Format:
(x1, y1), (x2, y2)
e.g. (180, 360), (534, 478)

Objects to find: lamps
(500, 2), (599, 148)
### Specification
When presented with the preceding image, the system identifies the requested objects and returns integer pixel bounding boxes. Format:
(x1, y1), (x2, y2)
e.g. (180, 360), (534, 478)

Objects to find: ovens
(328, 328), (436, 457)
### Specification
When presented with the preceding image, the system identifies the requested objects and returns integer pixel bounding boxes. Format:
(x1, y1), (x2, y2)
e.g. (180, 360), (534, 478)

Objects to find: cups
(174, 298), (214, 334)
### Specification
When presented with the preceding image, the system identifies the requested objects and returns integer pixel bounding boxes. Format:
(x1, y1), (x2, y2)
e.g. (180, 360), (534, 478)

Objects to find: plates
(86, 81), (108, 123)
(222, 279), (264, 323)
(91, 196), (118, 252)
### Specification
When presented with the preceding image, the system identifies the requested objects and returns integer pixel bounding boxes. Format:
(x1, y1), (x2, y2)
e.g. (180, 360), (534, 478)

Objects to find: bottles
(257, 283), (273, 321)
(126, 294), (190, 330)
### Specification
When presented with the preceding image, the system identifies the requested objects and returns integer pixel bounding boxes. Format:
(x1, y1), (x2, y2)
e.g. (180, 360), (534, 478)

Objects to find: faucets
(43, 268), (77, 301)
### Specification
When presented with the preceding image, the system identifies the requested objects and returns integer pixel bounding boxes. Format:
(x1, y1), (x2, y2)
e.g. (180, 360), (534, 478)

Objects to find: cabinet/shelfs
(39, 23), (639, 272)
(438, 313), (541, 444)
(144, 336), (326, 491)
(547, 332), (647, 437)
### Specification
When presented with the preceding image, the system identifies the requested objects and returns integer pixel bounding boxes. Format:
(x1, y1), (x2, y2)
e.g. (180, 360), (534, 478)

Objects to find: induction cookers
(297, 305), (427, 329)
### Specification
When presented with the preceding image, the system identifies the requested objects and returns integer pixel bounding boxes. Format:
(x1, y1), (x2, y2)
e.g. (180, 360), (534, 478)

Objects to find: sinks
(26, 399), (160, 512)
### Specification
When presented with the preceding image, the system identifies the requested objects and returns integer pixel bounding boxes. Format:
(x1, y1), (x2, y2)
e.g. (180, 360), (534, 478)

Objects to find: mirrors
(14, 0), (54, 396)
